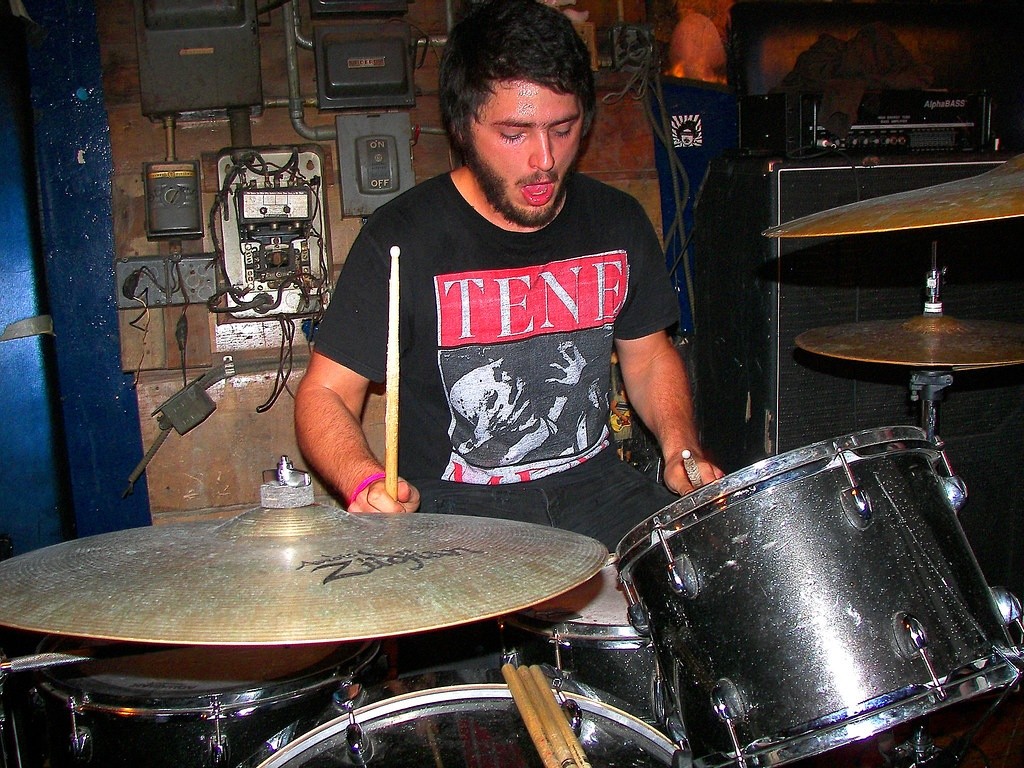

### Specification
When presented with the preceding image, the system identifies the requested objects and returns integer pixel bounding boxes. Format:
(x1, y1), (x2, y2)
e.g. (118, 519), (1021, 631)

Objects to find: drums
(253, 681), (687, 767)
(496, 548), (675, 728)
(32, 631), (387, 768)
(612, 423), (1023, 768)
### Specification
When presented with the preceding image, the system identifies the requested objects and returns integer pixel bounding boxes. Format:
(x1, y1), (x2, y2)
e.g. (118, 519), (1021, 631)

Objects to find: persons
(295, 0), (727, 671)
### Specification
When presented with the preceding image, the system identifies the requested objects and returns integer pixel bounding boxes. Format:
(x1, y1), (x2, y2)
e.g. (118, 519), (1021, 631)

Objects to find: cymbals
(793, 314), (1024, 367)
(0, 501), (609, 650)
(760, 150), (1024, 239)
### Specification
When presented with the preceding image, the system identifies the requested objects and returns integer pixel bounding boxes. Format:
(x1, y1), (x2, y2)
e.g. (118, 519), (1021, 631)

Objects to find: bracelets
(351, 471), (387, 504)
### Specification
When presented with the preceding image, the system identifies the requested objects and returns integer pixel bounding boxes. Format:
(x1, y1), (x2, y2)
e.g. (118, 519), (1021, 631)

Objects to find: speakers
(701, 152), (1023, 608)
